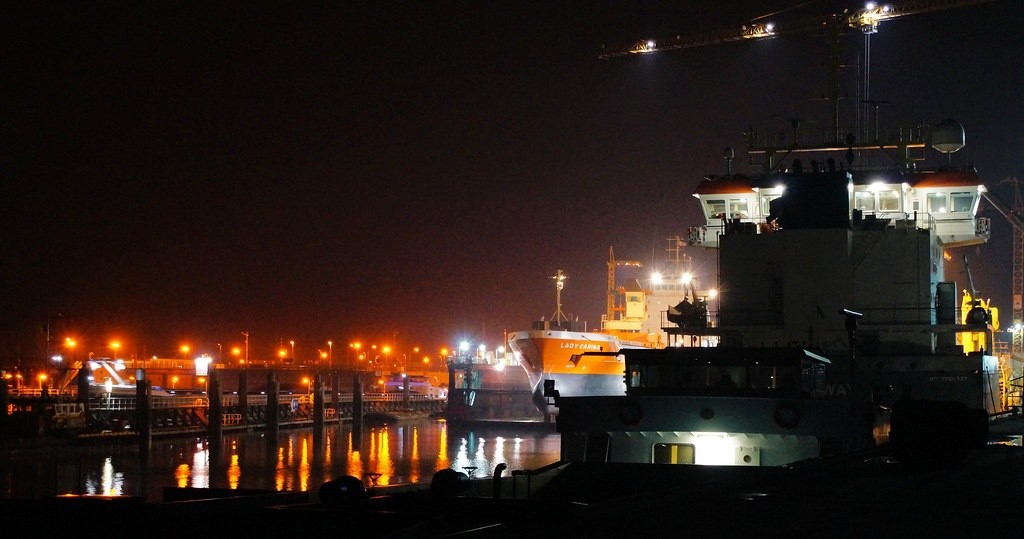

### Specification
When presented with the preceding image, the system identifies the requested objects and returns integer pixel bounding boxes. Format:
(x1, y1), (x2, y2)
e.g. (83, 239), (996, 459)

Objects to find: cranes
(592, 0), (980, 149)
(604, 246), (642, 320)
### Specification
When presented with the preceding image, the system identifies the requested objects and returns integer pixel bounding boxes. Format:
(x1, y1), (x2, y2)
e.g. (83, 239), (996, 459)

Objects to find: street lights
(353, 341), (362, 370)
(290, 340), (296, 369)
(328, 341), (334, 369)
(382, 345), (391, 364)
(180, 344), (189, 368)
(241, 331), (249, 365)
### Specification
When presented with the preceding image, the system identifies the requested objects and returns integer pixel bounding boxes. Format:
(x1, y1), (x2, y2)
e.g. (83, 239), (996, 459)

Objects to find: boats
(509, 318), (668, 415)
